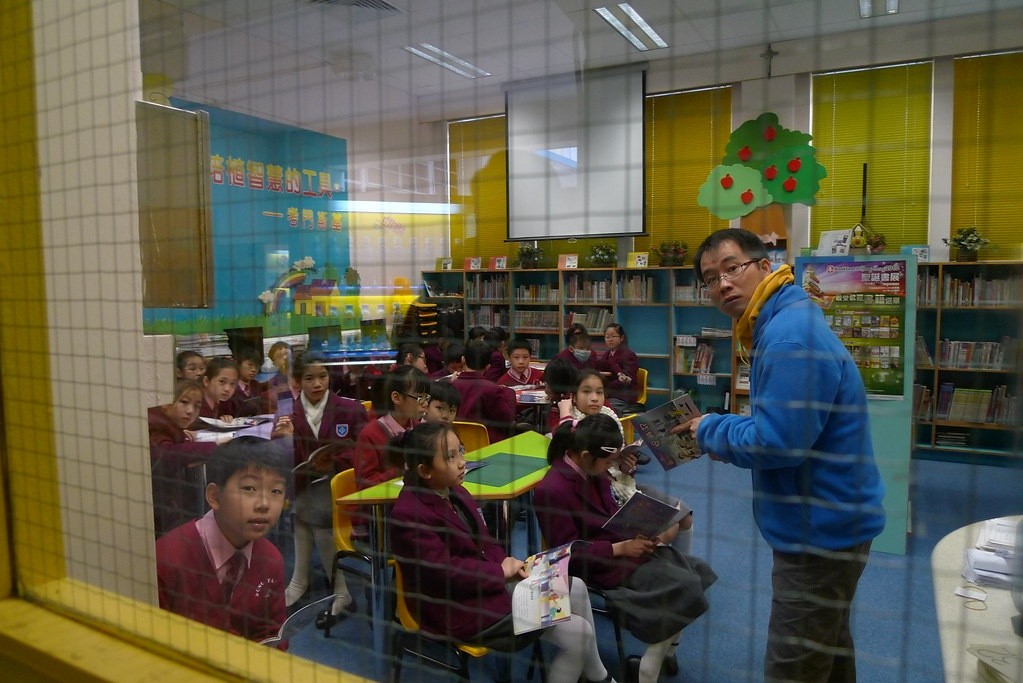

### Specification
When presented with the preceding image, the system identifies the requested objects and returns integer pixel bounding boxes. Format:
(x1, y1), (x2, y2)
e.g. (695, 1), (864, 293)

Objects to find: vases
(659, 256), (684, 266)
(851, 248), (868, 256)
(957, 249), (978, 262)
(522, 261), (537, 269)
(590, 262), (616, 268)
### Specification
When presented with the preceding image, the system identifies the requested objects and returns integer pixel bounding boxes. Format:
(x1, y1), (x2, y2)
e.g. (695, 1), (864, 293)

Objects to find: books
(936, 430), (970, 449)
(466, 273), (612, 361)
(600, 492), (686, 550)
(915, 332), (1022, 371)
(675, 279), (715, 303)
(198, 414), (286, 429)
(917, 267), (1023, 308)
(628, 395), (709, 471)
(616, 274), (653, 302)
(258, 592), (338, 647)
(511, 538), (593, 636)
(673, 341), (715, 374)
(915, 383), (1021, 426)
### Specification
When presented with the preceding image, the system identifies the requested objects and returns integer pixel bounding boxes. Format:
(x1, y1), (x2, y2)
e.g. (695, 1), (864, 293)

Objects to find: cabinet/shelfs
(421, 260), (1023, 469)
(731, 236), (791, 417)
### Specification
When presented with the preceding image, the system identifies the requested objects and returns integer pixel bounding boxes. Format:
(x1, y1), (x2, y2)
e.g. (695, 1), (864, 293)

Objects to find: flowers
(518, 243), (544, 264)
(586, 241), (617, 263)
(850, 230), (887, 254)
(650, 239), (688, 262)
(942, 226), (990, 251)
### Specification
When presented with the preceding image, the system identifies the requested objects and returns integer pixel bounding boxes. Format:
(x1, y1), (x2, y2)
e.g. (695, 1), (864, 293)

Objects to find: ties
(245, 385), (250, 396)
(214, 403), (219, 418)
(221, 552), (241, 609)
(442, 498), (454, 512)
(520, 374), (527, 382)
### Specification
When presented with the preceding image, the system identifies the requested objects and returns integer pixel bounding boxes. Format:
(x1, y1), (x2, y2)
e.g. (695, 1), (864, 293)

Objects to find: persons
(155, 435), (289, 653)
(385, 420), (619, 683)
(533, 413), (718, 682)
(172, 339), (293, 429)
(556, 367), (693, 560)
(270, 349), (368, 628)
(148, 381), (219, 465)
(352, 364), (459, 566)
(669, 228), (886, 683)
(398, 321), (641, 521)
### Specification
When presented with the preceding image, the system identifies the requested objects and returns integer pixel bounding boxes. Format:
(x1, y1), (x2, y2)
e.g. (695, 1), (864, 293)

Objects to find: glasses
(411, 353), (426, 363)
(604, 334), (621, 340)
(701, 257), (761, 291)
(408, 393), (431, 404)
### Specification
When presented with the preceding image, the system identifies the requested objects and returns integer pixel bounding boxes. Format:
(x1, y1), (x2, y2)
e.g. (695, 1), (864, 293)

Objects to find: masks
(571, 348), (592, 362)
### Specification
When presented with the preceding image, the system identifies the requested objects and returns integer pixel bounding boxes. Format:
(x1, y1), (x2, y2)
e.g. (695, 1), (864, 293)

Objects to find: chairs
(327, 369), (648, 683)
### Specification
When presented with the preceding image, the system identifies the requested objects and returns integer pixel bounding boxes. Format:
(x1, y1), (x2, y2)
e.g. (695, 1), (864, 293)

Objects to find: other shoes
(663, 653), (679, 676)
(315, 597), (357, 628)
(285, 588), (312, 616)
(635, 451), (650, 464)
(626, 654), (642, 683)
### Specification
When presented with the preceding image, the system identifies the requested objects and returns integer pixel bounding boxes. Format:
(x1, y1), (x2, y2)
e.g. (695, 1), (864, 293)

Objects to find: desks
(931, 515), (1023, 682)
(515, 387), (553, 435)
(335, 430), (552, 682)
(185, 397), (366, 519)
